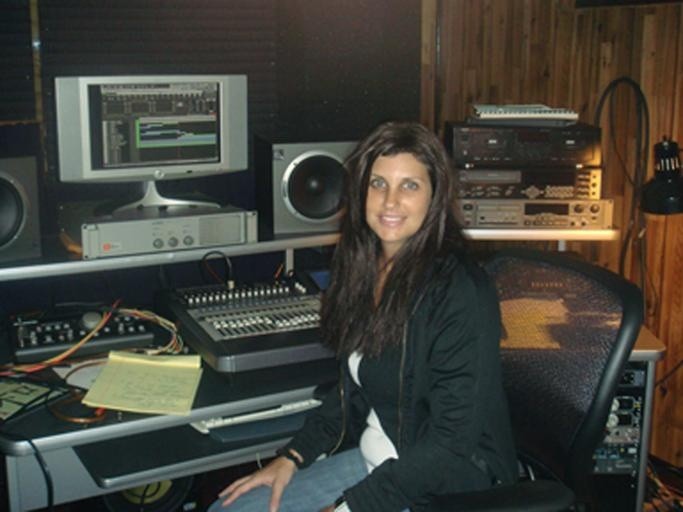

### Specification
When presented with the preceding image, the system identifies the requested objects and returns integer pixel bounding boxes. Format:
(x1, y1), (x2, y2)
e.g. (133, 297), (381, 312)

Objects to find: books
(80, 349), (203, 416)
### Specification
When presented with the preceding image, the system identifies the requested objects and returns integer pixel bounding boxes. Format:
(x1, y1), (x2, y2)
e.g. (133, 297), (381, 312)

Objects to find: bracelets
(275, 445), (301, 471)
(334, 495), (344, 507)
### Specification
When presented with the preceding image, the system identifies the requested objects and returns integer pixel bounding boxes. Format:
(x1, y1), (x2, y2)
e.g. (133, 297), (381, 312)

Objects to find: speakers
(266, 136), (361, 237)
(0, 156), (43, 268)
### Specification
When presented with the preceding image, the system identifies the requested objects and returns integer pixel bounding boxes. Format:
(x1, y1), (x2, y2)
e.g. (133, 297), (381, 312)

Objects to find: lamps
(640, 135), (683, 216)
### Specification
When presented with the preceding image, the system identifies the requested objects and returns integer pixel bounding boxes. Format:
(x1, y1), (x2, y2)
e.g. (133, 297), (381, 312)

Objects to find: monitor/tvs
(53, 75), (249, 208)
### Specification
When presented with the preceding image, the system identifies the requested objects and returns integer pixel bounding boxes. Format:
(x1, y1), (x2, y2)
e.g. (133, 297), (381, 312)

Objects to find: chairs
(423, 247), (645, 510)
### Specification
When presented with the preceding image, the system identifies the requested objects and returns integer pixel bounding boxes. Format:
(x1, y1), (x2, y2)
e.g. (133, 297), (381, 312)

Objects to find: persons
(201, 118), (519, 512)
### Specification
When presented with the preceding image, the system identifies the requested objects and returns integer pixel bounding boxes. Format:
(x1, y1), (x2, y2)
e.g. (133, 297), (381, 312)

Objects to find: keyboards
(188, 398), (324, 433)
